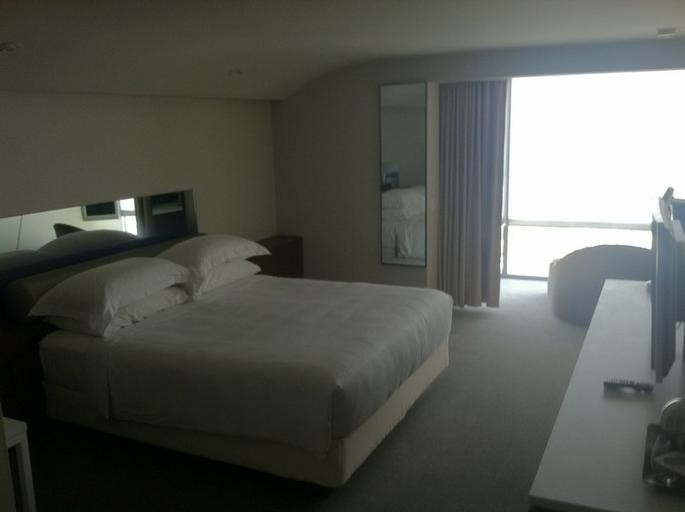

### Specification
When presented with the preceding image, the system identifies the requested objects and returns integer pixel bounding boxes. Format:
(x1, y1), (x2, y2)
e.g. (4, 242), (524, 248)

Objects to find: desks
(0, 416), (36, 511)
(528, 280), (685, 512)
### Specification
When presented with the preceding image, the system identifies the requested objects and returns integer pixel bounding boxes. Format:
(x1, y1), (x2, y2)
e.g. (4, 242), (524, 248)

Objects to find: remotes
(603, 377), (654, 393)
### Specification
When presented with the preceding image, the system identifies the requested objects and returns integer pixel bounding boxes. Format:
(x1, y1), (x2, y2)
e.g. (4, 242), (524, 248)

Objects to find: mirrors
(379, 81), (427, 269)
(0, 193), (186, 271)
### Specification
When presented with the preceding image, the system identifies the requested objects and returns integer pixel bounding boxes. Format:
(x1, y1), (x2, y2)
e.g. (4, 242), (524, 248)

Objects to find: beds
(380, 184), (426, 266)
(0, 231), (455, 488)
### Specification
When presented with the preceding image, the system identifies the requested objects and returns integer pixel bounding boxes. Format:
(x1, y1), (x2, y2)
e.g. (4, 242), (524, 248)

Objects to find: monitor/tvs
(151, 191), (184, 216)
(658, 187), (685, 220)
(81, 200), (121, 221)
(650, 215), (685, 384)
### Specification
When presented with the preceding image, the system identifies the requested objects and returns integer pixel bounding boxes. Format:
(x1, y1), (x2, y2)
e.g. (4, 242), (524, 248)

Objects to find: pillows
(178, 260), (261, 302)
(381, 188), (423, 209)
(155, 231), (272, 279)
(42, 285), (188, 338)
(381, 207), (423, 219)
(27, 256), (190, 321)
(0, 249), (58, 273)
(37, 230), (141, 263)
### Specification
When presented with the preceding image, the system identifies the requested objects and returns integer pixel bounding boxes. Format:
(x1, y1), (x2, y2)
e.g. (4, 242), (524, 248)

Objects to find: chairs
(53, 223), (83, 238)
(547, 245), (652, 327)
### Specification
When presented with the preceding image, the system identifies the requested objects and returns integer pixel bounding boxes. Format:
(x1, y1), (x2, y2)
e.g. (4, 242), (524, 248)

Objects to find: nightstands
(257, 233), (304, 276)
